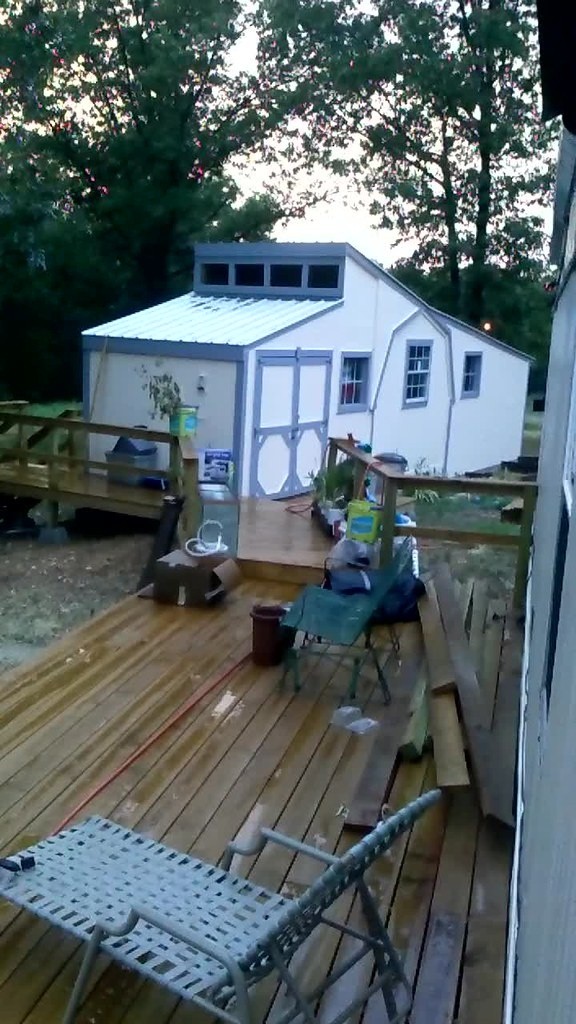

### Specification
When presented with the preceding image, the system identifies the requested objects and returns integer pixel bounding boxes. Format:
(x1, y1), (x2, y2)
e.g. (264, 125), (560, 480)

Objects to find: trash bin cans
(251, 605), (298, 666)
(373, 452), (408, 495)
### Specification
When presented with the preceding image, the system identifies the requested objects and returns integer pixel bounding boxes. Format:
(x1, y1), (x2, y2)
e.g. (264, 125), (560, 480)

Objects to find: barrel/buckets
(249, 602), (294, 667)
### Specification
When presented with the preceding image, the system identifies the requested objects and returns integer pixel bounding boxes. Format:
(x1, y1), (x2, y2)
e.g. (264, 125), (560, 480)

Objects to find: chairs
(1, 789), (444, 1024)
(279, 531), (414, 705)
